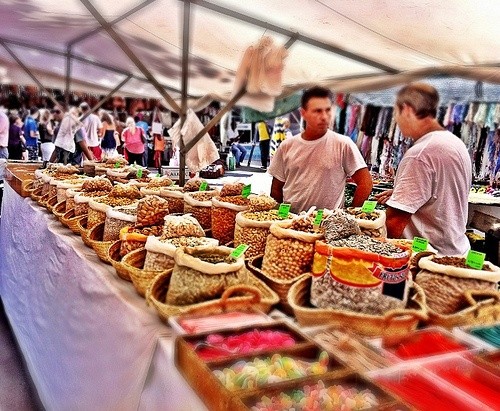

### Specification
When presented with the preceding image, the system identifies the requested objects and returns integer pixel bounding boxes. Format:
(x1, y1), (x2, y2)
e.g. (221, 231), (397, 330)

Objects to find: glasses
(50, 110), (61, 114)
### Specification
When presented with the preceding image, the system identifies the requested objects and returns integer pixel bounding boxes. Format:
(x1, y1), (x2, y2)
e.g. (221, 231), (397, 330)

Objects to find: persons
(252, 122), (270, 170)
(270, 86), (372, 209)
(283, 118), (292, 139)
(375, 85), (471, 259)
(0, 100), (148, 168)
(121, 118), (146, 167)
(227, 121), (247, 168)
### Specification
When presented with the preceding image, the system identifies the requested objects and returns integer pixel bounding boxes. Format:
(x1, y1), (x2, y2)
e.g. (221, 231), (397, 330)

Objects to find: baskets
(24, 164), (308, 327)
(410, 251), (500, 333)
(286, 273), (429, 346)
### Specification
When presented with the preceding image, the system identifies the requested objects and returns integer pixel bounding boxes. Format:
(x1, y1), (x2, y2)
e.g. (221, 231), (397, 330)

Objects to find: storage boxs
(174, 320), (353, 411)
(228, 371), (418, 411)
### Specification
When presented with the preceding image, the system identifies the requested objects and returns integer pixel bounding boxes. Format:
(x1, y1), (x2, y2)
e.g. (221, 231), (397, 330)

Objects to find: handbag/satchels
(226, 152), (236, 170)
(114, 131), (121, 148)
(139, 127), (146, 143)
